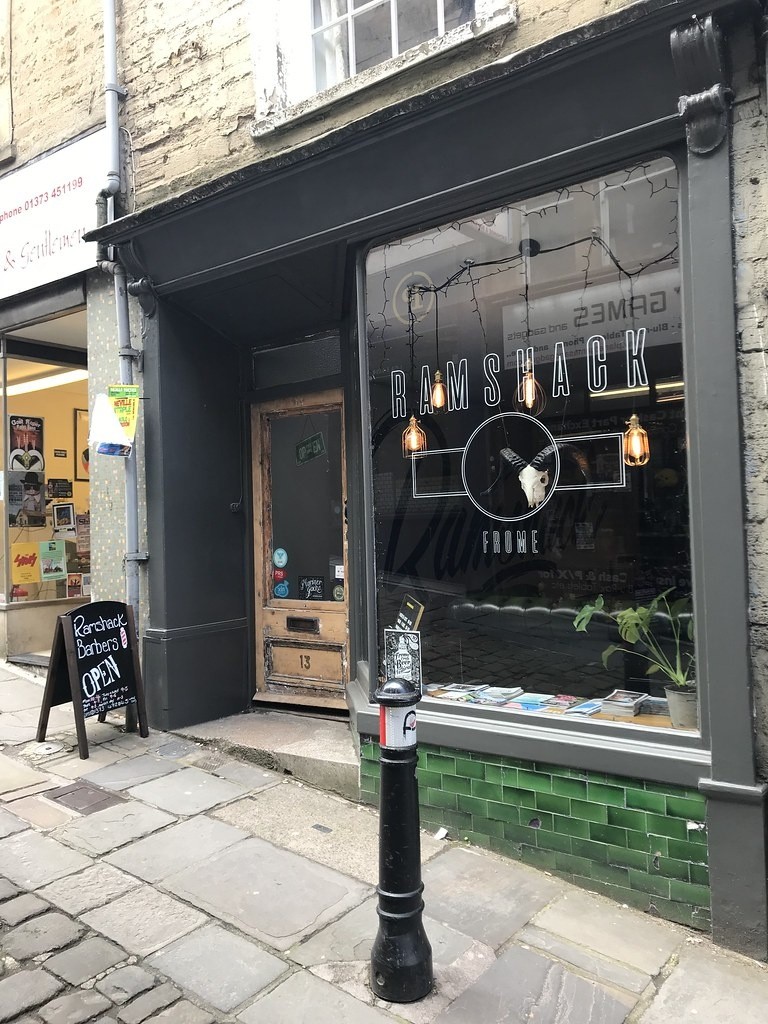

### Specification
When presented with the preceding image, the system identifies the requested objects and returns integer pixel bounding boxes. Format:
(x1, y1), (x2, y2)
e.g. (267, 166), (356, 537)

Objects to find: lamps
(621, 275), (650, 467)
(502, 239), (547, 418)
(403, 286), (427, 459)
(426, 284), (450, 414)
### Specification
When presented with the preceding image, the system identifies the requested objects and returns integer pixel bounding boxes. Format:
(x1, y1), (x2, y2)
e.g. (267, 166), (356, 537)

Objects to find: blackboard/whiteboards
(42, 601), (145, 720)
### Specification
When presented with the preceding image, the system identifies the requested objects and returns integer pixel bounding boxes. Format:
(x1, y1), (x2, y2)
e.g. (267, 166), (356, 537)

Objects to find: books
(437, 683), (670, 718)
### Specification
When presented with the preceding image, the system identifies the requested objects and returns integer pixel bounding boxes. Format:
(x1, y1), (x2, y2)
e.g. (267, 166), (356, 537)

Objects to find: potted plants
(573, 586), (697, 733)
